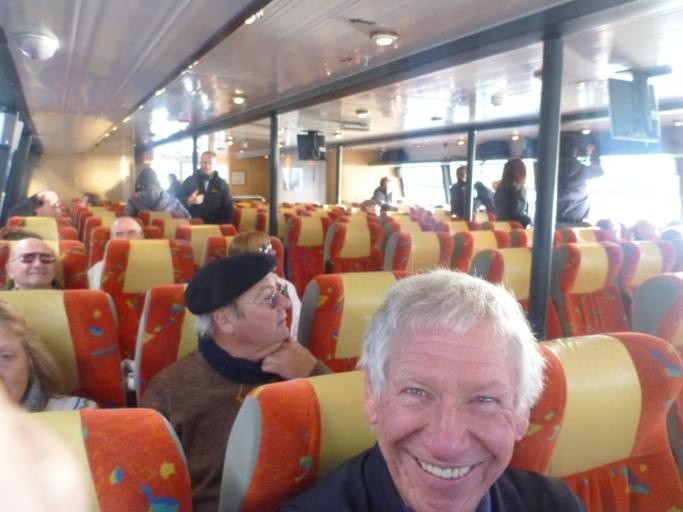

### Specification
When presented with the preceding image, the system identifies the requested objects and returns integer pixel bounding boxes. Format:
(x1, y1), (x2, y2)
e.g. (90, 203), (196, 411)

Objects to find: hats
(182, 250), (279, 316)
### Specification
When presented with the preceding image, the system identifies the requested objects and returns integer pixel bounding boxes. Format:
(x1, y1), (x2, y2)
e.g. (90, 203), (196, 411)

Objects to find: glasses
(9, 253), (56, 265)
(223, 286), (288, 311)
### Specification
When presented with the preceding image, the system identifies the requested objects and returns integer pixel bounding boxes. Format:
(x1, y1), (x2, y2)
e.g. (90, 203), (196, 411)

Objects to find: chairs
(0, 198), (683, 509)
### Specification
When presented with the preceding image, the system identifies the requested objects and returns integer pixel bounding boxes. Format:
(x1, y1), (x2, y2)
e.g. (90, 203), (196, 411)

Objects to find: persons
(228, 231), (302, 341)
(124, 151), (234, 224)
(141, 252), (333, 510)
(0, 191), (144, 413)
(277, 267), (588, 511)
(360, 138), (603, 225)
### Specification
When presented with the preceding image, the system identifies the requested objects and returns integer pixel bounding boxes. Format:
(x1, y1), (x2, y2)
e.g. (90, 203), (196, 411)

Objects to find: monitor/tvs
(296, 134), (326, 161)
(607, 78), (661, 144)
